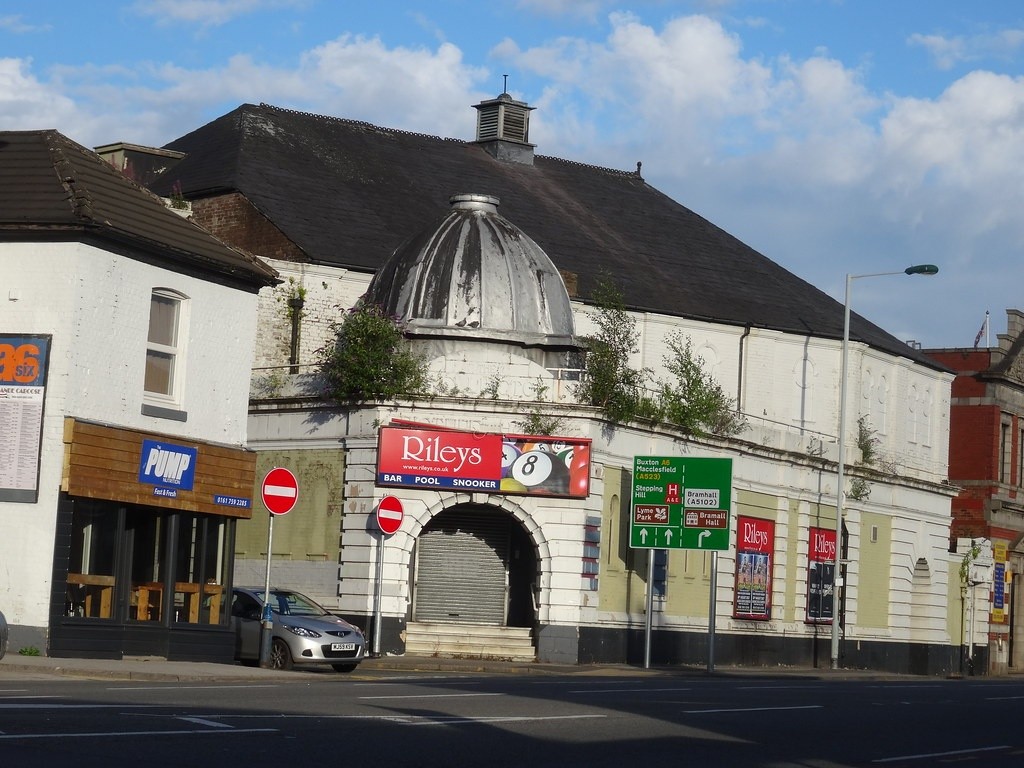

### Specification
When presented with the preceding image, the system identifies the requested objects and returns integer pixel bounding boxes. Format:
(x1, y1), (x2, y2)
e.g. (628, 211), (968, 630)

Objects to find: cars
(201, 585), (367, 672)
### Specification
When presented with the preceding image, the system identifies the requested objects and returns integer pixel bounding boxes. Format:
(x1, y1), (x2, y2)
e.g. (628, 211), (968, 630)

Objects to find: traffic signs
(629, 454), (732, 549)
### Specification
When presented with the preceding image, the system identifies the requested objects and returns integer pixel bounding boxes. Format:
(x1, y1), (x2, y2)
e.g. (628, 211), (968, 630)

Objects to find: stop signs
(262, 468), (300, 516)
(377, 496), (404, 535)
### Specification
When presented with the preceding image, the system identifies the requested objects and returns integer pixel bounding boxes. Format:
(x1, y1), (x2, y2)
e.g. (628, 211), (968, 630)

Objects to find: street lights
(831, 265), (938, 672)
